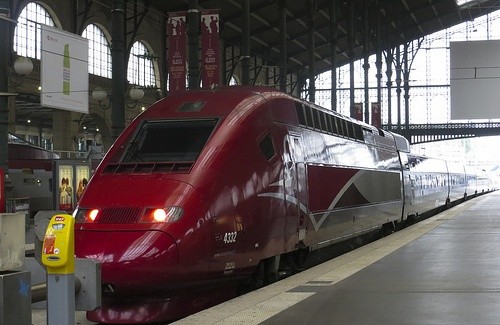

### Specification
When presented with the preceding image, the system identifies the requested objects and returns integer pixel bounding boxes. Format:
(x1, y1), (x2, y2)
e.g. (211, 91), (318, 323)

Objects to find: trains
(67, 86), (499, 325)
